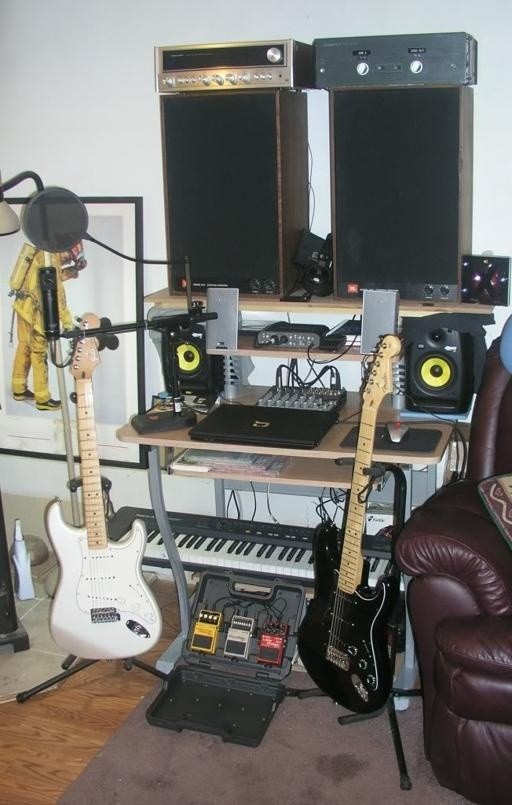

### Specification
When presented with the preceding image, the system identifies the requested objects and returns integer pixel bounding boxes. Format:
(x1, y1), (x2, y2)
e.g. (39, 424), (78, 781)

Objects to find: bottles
(9, 520), (35, 601)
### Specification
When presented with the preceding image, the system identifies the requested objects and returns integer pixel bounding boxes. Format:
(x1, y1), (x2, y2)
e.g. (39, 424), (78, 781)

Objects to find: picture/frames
(0, 197), (150, 471)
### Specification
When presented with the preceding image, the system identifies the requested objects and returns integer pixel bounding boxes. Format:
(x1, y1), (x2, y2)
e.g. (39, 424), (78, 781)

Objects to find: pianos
(104, 505), (407, 592)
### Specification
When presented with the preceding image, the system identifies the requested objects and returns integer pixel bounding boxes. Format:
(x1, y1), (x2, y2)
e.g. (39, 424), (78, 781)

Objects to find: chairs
(392, 338), (512, 804)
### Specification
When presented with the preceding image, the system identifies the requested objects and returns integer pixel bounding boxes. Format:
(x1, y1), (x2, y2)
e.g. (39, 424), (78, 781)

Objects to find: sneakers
(36, 398), (61, 410)
(14, 390), (34, 401)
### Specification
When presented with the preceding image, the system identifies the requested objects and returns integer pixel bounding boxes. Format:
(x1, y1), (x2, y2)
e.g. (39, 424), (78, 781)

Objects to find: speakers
(330, 86), (474, 306)
(162, 325), (223, 394)
(404, 315), (474, 414)
(159, 90), (309, 300)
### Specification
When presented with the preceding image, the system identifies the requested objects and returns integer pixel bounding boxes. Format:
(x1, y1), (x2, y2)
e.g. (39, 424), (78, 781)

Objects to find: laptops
(188, 403), (339, 449)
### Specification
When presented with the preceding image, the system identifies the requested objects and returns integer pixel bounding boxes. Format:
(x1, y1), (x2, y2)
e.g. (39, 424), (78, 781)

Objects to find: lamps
(1, 170), (87, 528)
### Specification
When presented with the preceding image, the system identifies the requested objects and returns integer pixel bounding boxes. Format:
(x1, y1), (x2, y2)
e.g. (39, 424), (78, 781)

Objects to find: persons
(12, 238), (85, 411)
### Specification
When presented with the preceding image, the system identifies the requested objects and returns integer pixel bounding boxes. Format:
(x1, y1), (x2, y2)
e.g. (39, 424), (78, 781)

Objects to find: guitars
(297, 335), (400, 715)
(44, 314), (162, 659)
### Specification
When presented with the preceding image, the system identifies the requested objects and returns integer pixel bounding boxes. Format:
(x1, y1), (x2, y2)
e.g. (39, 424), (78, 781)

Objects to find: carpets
(57, 666), (462, 803)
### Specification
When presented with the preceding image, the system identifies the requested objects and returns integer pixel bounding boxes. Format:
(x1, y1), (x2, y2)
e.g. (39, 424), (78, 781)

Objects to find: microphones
(39, 265), (61, 341)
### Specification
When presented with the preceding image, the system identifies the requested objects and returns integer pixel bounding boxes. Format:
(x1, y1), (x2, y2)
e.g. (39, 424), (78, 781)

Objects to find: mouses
(384, 422), (410, 444)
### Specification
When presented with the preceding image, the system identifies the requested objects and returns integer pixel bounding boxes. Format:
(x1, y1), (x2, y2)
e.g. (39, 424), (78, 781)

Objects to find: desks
(117, 287), (497, 712)
